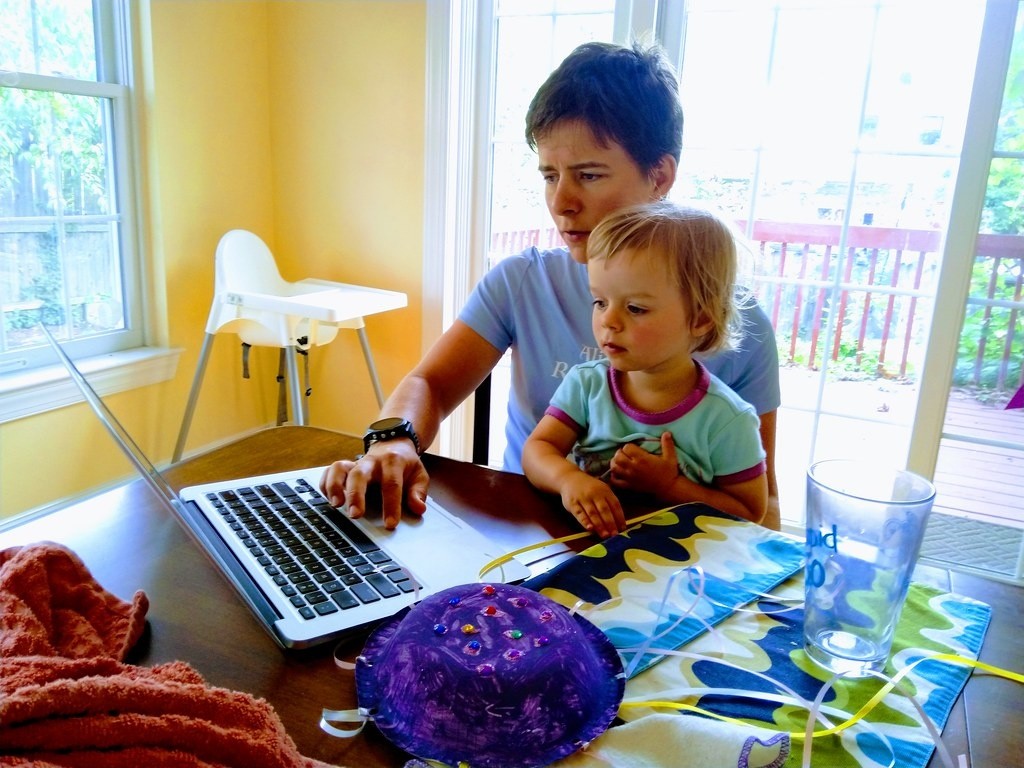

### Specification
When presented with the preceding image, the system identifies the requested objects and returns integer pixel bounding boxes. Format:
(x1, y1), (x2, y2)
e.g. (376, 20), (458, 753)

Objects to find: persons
(521, 204), (769, 539)
(319, 42), (781, 532)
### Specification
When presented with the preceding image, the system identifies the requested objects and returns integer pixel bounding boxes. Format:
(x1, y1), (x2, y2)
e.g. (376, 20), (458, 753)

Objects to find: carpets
(919, 509), (1024, 579)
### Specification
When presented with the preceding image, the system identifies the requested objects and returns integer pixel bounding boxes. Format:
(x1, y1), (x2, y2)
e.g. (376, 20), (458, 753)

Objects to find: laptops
(36, 320), (533, 652)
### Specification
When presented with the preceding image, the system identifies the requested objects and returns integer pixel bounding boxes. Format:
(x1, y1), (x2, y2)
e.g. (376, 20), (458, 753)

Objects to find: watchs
(362, 417), (421, 456)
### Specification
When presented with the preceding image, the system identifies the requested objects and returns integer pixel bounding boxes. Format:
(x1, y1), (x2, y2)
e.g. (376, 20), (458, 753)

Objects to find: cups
(805, 458), (937, 678)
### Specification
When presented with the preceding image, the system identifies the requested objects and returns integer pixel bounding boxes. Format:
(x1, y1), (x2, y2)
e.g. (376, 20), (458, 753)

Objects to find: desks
(949, 571), (1024, 768)
(0, 427), (968, 768)
(224, 277), (408, 322)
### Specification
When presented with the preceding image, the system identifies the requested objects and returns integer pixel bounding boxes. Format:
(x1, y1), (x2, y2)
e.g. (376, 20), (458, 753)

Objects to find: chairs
(171, 229), (384, 465)
